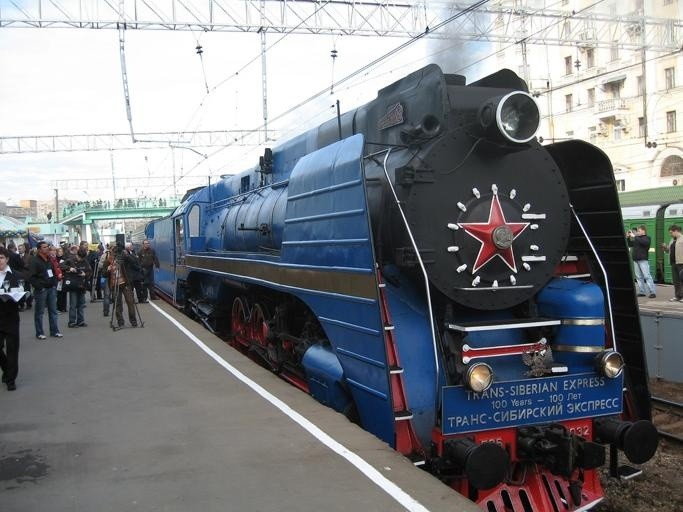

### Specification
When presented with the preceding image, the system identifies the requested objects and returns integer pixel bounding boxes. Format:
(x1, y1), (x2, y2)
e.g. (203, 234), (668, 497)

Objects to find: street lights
(54, 187), (60, 224)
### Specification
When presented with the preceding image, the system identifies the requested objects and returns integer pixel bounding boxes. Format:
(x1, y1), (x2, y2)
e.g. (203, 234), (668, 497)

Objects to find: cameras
(76, 267), (82, 274)
(627, 231), (631, 233)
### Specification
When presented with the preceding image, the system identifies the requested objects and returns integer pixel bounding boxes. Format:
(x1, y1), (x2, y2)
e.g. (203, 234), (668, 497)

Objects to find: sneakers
(79, 322), (87, 327)
(36, 334), (47, 340)
(54, 333), (63, 337)
(68, 323), (78, 328)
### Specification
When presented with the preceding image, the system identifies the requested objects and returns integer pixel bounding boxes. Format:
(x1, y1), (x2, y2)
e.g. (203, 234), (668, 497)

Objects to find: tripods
(90, 263), (106, 303)
(109, 264), (145, 332)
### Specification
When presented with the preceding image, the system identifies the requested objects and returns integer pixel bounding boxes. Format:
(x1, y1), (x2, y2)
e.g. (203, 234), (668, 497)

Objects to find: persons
(626, 225), (656, 298)
(662, 225), (683, 303)
(0, 196), (166, 390)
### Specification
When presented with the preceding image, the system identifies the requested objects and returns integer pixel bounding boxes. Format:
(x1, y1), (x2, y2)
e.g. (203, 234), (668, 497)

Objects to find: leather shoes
(2, 371), (9, 383)
(6, 380), (16, 391)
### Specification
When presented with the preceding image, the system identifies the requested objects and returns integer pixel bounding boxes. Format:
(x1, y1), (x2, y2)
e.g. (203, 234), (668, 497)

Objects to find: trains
(128, 64), (659, 512)
(617, 186), (682, 283)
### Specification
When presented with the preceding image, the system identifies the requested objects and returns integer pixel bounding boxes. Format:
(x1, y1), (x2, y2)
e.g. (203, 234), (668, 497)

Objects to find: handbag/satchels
(64, 271), (82, 288)
